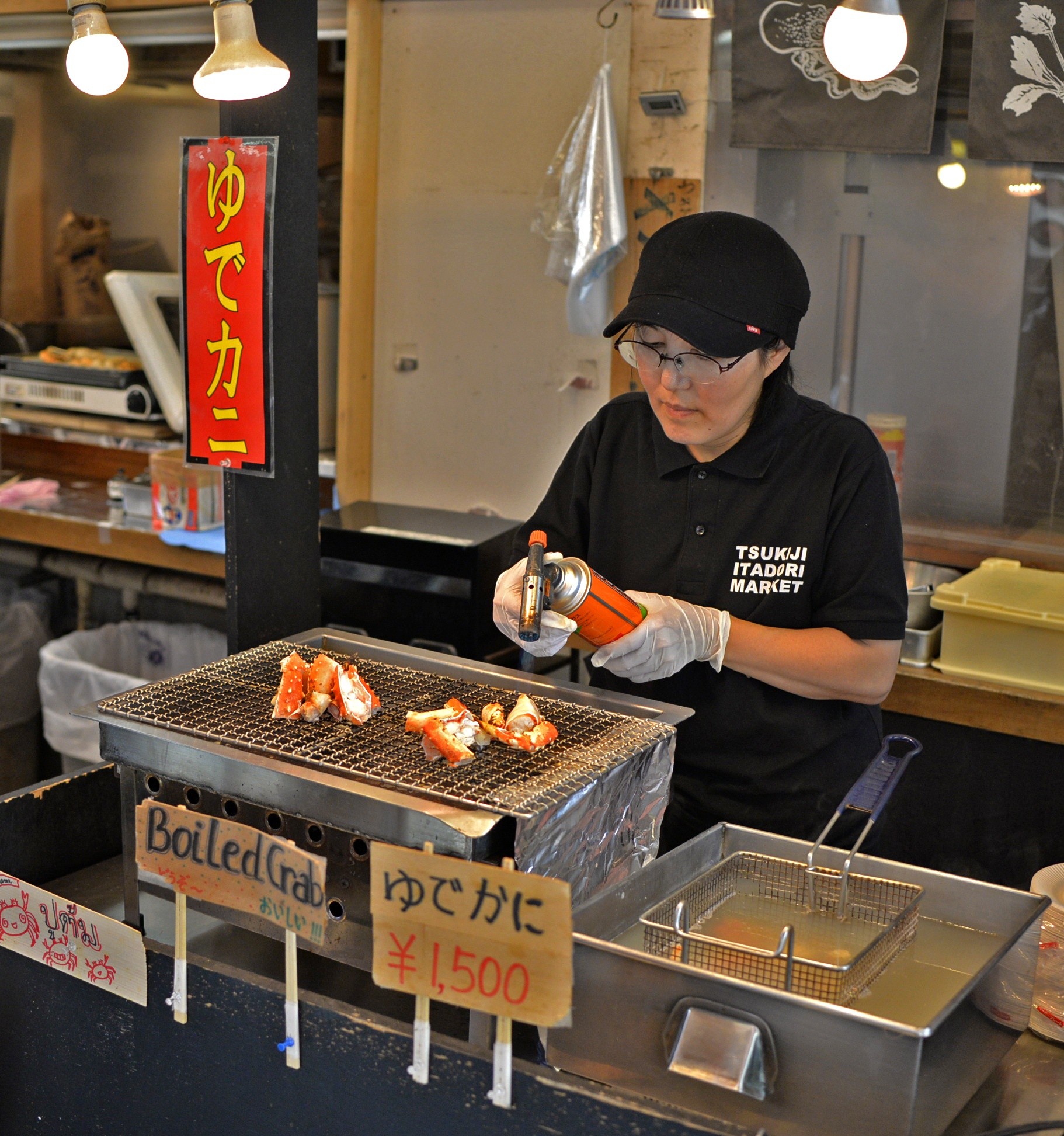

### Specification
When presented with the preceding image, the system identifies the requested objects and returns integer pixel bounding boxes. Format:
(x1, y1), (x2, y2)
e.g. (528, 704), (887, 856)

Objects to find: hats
(603, 211), (811, 358)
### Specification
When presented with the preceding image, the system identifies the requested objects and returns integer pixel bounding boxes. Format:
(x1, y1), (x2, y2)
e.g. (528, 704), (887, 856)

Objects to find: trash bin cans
(38, 619), (229, 774)
(0, 574), (50, 795)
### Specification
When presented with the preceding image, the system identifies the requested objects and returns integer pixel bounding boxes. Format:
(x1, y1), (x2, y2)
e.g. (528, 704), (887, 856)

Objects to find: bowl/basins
(905, 559), (963, 627)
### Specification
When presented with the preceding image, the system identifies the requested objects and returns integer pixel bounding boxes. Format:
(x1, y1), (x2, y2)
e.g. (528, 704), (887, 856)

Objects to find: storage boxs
(929, 555), (1064, 697)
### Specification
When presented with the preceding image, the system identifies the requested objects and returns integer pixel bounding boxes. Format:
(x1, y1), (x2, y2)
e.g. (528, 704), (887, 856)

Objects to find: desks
(878, 654), (1064, 754)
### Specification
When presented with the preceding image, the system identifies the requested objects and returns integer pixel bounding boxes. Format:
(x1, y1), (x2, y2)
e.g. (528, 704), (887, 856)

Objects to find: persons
(493, 211), (908, 878)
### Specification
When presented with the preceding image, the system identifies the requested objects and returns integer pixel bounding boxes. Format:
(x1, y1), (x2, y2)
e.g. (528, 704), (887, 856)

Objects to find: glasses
(614, 322), (750, 385)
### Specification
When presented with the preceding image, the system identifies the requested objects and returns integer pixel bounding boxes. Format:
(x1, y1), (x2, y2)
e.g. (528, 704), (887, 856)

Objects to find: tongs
(908, 583), (933, 592)
